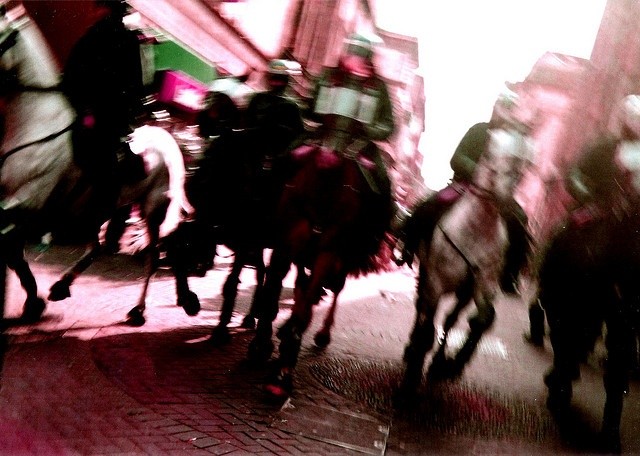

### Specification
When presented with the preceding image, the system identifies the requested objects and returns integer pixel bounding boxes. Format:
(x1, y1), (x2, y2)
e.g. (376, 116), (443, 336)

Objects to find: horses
(395, 128), (535, 413)
(245, 139), (397, 404)
(539, 202), (640, 454)
(160, 92), (276, 342)
(0, 7), (200, 324)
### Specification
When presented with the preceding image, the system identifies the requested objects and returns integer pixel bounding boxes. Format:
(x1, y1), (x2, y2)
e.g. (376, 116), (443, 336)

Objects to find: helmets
(613, 95), (640, 139)
(266, 59), (291, 75)
(342, 34), (373, 59)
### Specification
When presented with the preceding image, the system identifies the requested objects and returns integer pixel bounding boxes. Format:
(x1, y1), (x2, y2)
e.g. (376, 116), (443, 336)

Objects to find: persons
(408, 95), (529, 297)
(238, 60), (290, 205)
(1, 0), (163, 180)
(566, 95), (640, 231)
(308, 34), (393, 209)
